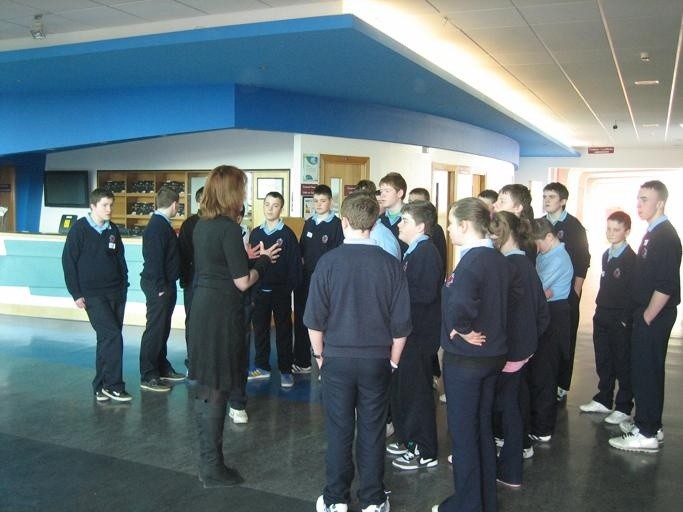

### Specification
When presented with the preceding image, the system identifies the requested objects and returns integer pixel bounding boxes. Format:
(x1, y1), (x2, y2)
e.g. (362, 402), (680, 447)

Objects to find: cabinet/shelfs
(97, 169), (189, 235)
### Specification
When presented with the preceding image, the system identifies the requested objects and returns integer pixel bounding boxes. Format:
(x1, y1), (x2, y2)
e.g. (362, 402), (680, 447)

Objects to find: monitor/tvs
(42, 169), (90, 208)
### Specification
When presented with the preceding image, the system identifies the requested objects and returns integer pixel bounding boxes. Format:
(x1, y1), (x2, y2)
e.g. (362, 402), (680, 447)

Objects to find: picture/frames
(302, 197), (313, 218)
(257, 177), (284, 200)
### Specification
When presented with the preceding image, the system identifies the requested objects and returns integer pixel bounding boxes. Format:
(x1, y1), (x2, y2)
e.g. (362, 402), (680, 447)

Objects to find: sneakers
(247, 368), (271, 379)
(140, 370), (185, 392)
(229, 407), (248, 424)
(96, 387), (132, 401)
(494, 435), (552, 487)
(199, 463), (242, 487)
(386, 422), (438, 470)
(579, 400), (663, 453)
(280, 364), (312, 387)
(315, 495), (390, 512)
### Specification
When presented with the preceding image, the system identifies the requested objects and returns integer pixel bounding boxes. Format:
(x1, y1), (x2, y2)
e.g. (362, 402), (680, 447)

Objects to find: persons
(139, 185), (185, 392)
(580, 211), (638, 424)
(609, 180), (683, 452)
(431, 180), (591, 511)
(247, 171), (448, 511)
(61, 188), (133, 400)
(187, 165), (283, 489)
(227, 206), (250, 424)
(177, 186), (203, 374)
